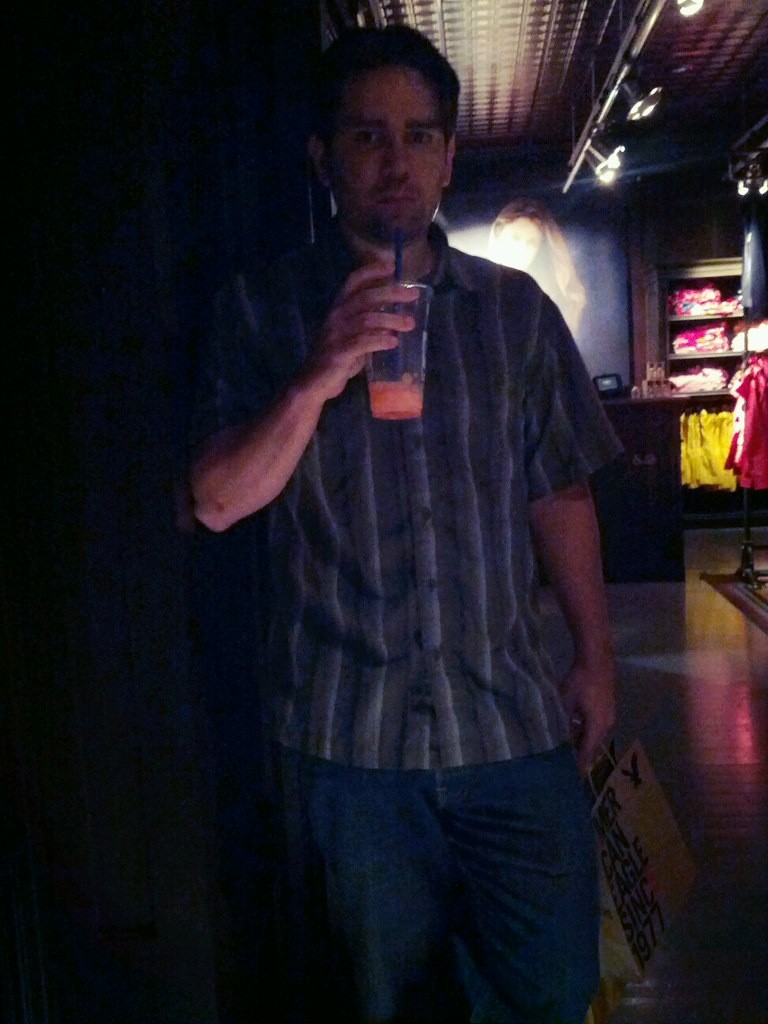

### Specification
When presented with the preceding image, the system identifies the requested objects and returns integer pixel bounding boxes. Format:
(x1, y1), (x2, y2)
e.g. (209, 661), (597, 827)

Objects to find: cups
(361, 284), (432, 418)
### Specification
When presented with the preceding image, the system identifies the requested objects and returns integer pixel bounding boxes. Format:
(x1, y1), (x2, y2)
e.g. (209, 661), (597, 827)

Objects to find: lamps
(583, 130), (625, 178)
(618, 74), (663, 121)
(676, 0), (706, 16)
(592, 369), (623, 394)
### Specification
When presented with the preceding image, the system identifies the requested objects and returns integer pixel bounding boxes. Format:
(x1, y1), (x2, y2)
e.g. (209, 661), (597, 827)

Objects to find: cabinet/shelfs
(663, 253), (768, 383)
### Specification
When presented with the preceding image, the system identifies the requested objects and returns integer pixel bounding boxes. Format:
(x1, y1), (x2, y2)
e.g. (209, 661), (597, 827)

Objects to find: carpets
(701, 570), (768, 636)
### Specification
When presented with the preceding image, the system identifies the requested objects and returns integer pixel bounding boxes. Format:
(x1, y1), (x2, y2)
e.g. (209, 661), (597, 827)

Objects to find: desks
(675, 391), (768, 527)
(588, 392), (688, 580)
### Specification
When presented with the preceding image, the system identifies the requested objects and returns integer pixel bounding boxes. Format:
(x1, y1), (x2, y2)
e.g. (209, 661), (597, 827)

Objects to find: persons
(489, 198), (585, 341)
(188, 24), (627, 1023)
